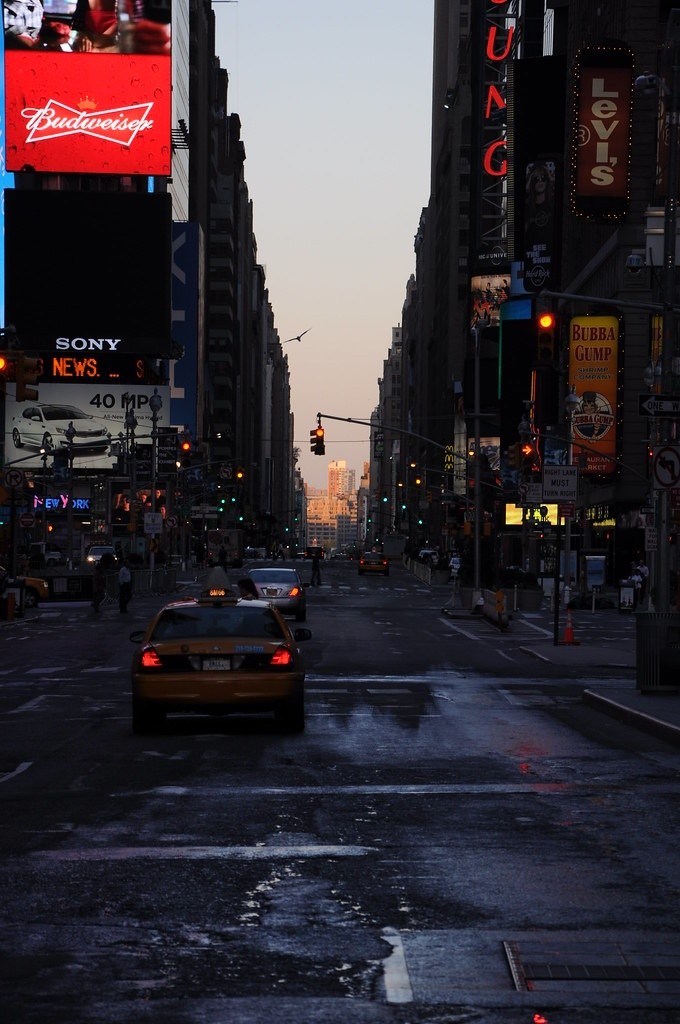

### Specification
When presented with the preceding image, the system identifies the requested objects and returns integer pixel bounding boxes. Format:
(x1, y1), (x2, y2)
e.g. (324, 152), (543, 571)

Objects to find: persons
(91, 565), (107, 613)
(310, 550), (322, 587)
(480, 279), (510, 298)
(118, 559), (132, 613)
(237, 578), (258, 599)
(275, 541), (285, 560)
(218, 545), (228, 572)
(628, 560), (649, 611)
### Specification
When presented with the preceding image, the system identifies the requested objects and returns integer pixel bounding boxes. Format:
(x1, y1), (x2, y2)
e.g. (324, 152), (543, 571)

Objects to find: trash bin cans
(636, 614), (679, 698)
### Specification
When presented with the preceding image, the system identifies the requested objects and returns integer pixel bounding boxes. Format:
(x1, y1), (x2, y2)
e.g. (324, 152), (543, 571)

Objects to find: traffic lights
(310, 429), (326, 455)
(178, 440), (193, 470)
(521, 444), (534, 458)
(534, 314), (559, 365)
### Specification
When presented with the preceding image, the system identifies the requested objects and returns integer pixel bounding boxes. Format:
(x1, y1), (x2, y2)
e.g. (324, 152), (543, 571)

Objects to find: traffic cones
(558, 608), (581, 645)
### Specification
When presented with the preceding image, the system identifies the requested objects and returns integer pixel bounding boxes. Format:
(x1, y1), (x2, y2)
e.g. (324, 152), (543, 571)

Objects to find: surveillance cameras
(626, 254), (643, 273)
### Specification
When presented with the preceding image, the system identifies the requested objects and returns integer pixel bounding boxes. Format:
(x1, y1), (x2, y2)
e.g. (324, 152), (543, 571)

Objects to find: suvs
(304, 546), (324, 559)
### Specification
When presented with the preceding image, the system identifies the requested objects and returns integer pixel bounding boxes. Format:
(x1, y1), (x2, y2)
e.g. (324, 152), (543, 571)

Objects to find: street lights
(149, 387), (163, 586)
(415, 479), (483, 614)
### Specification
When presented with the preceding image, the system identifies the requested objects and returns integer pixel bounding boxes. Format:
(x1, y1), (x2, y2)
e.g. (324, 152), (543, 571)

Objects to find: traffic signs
(637, 392), (680, 418)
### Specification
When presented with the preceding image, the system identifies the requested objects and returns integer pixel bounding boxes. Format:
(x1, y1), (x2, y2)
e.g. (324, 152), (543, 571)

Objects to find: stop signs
(19, 512), (36, 528)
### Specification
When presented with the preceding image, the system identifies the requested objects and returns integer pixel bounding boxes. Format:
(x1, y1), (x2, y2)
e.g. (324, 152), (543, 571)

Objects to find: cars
(448, 557), (465, 577)
(0, 565), (49, 608)
(86, 546), (119, 567)
(419, 550), (440, 565)
(11, 403), (112, 456)
(246, 567), (307, 622)
(129, 587), (313, 735)
(358, 546), (390, 576)
